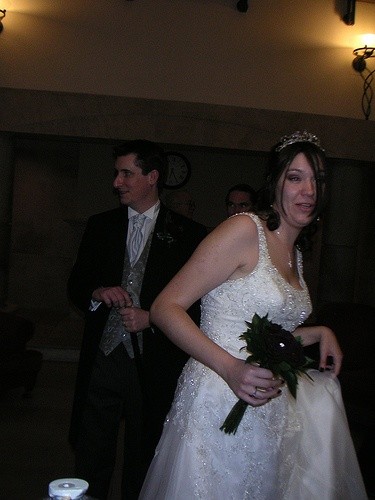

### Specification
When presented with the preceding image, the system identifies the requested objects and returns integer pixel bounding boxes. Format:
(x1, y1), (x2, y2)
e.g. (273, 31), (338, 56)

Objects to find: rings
(249, 389), (257, 398)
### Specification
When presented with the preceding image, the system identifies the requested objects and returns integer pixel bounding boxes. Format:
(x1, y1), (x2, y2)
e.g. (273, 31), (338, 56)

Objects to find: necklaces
(275, 230), (295, 268)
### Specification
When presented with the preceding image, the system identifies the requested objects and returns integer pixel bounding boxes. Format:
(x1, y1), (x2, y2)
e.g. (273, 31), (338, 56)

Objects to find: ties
(126, 214), (147, 267)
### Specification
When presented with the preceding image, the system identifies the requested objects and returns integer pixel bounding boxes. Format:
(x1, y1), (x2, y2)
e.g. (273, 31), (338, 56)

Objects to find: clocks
(160, 151), (192, 190)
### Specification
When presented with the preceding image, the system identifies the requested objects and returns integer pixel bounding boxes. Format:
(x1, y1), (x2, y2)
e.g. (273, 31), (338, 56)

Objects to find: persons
(148, 127), (345, 500)
(167, 189), (199, 218)
(225, 184), (256, 217)
(66, 142), (203, 499)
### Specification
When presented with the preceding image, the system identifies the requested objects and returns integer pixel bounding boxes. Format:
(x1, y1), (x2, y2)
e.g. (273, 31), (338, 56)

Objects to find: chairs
(0, 312), (43, 399)
(316, 302), (375, 452)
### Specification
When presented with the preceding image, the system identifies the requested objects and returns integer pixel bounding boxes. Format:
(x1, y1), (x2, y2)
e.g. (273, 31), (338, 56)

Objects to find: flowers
(219, 312), (314, 436)
(156, 230), (177, 250)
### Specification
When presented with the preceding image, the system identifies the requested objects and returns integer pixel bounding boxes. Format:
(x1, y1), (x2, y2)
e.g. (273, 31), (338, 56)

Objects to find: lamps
(351, 33), (375, 72)
